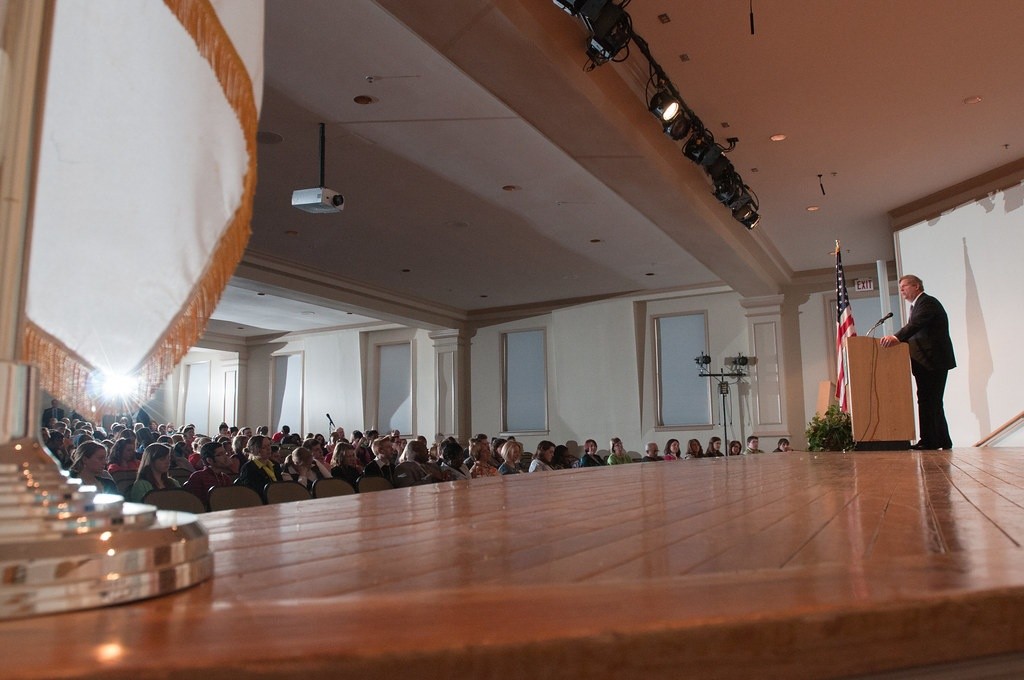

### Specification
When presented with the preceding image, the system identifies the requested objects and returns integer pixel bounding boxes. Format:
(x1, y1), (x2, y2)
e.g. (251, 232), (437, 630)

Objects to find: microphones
(326, 413), (335, 426)
(878, 312), (893, 323)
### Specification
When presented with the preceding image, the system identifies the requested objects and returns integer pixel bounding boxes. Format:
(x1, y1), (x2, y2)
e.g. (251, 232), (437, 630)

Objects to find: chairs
(355, 475), (394, 493)
(519, 451), (533, 473)
(312, 478), (357, 498)
(566, 440), (584, 459)
(140, 488), (205, 514)
(627, 451), (642, 462)
(115, 467), (192, 489)
(596, 449), (609, 459)
(263, 480), (314, 505)
(208, 484), (264, 512)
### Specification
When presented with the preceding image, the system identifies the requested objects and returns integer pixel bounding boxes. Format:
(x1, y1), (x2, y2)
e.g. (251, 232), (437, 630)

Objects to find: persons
(728, 441), (742, 455)
(606, 438), (633, 465)
(705, 437), (724, 457)
(662, 439), (681, 460)
(42, 418), (522, 503)
(43, 399), (65, 427)
(641, 442), (664, 462)
(684, 439), (704, 459)
(742, 436), (765, 454)
(880, 275), (957, 451)
(581, 440), (605, 467)
(130, 407), (149, 429)
(773, 438), (793, 452)
(529, 441), (572, 473)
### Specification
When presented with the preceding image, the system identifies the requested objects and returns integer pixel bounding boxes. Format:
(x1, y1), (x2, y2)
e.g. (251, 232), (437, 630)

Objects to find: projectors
(291, 187), (345, 213)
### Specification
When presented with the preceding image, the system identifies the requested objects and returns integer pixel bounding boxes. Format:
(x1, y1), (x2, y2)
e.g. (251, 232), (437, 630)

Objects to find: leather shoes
(910, 441), (952, 451)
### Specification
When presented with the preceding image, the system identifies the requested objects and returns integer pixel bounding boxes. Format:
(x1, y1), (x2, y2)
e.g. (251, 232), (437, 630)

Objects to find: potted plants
(804, 402), (857, 453)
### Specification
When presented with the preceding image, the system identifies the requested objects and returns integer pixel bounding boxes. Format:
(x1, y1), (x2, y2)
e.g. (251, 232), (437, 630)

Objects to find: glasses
(211, 451), (226, 457)
(649, 449), (659, 452)
(899, 285), (913, 290)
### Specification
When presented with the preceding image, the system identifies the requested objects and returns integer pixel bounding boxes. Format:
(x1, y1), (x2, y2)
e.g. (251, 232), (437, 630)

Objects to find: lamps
(733, 357), (748, 366)
(648, 90), (761, 228)
(695, 355), (711, 364)
(553, 0), (632, 64)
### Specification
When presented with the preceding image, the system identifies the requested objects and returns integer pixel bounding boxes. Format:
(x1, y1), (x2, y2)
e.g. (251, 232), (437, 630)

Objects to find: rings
(886, 340), (888, 342)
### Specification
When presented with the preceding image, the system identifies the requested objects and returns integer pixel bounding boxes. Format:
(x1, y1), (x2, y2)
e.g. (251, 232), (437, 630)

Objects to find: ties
(54, 409), (56, 418)
(909, 305), (913, 319)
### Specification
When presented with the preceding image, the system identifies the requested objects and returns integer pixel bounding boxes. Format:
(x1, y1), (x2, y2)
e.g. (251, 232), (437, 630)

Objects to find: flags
(834, 247), (857, 412)
